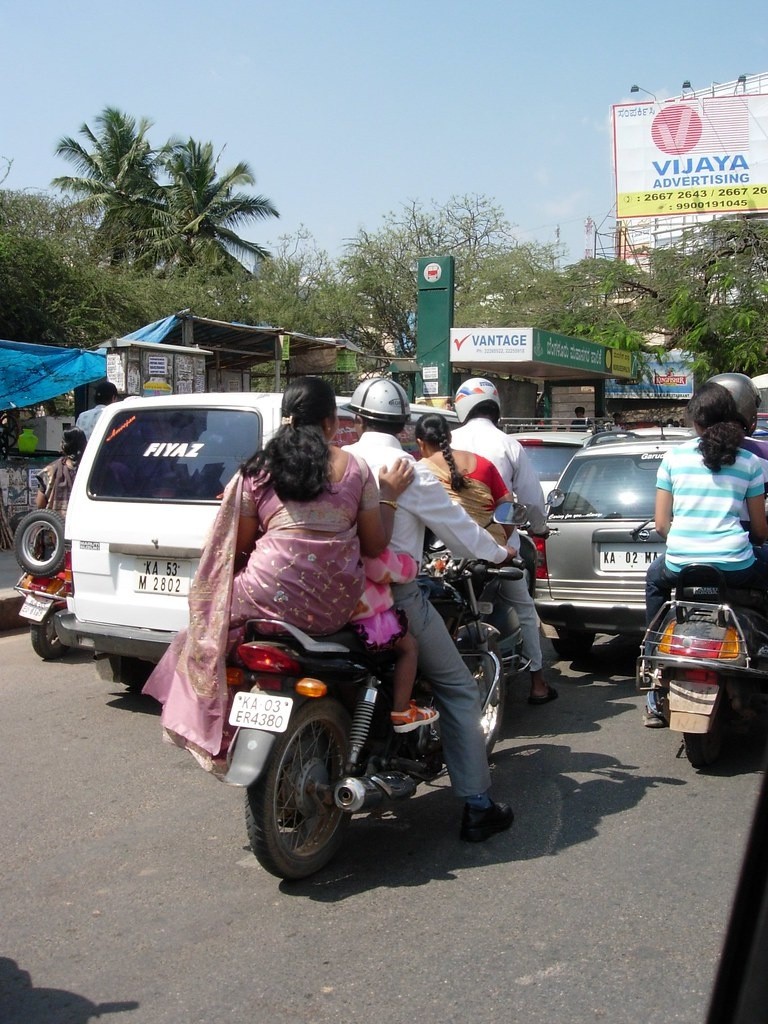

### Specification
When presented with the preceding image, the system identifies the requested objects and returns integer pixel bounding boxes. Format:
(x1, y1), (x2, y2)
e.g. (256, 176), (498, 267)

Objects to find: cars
(499, 427), (699, 660)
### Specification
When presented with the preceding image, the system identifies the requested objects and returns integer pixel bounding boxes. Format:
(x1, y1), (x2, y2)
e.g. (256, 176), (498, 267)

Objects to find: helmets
(452, 377), (502, 426)
(707, 359), (762, 436)
(338, 377), (413, 424)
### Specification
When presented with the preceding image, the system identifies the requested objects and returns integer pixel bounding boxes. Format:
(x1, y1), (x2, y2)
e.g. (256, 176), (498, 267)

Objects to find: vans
(65, 391), (462, 696)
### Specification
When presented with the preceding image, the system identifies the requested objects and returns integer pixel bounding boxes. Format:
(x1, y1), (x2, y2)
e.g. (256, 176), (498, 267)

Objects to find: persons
(35, 382), (119, 559)
(140, 373), (416, 783)
(555, 408), (686, 437)
(338, 377), (518, 845)
(642, 373), (768, 728)
(353, 550), (440, 735)
(413, 378), (559, 707)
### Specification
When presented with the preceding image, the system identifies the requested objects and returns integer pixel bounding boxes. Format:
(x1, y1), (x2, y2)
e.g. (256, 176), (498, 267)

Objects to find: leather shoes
(461, 799), (519, 842)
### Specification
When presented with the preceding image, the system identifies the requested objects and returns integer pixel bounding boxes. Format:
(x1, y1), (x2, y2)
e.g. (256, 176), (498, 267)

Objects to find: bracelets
(378, 500), (397, 510)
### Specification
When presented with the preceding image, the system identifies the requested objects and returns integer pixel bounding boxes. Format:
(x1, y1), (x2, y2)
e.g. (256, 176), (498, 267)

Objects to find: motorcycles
(226, 487), (566, 882)
(12, 506), (73, 660)
(635, 555), (767, 770)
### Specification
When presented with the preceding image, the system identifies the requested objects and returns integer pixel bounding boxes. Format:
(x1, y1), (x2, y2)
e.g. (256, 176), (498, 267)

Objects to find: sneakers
(642, 709), (668, 729)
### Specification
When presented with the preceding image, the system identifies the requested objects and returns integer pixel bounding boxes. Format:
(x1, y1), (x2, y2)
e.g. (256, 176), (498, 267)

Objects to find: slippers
(521, 684), (562, 705)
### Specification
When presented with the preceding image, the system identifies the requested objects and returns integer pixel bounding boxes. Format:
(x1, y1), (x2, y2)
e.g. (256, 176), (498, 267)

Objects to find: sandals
(391, 701), (440, 734)
